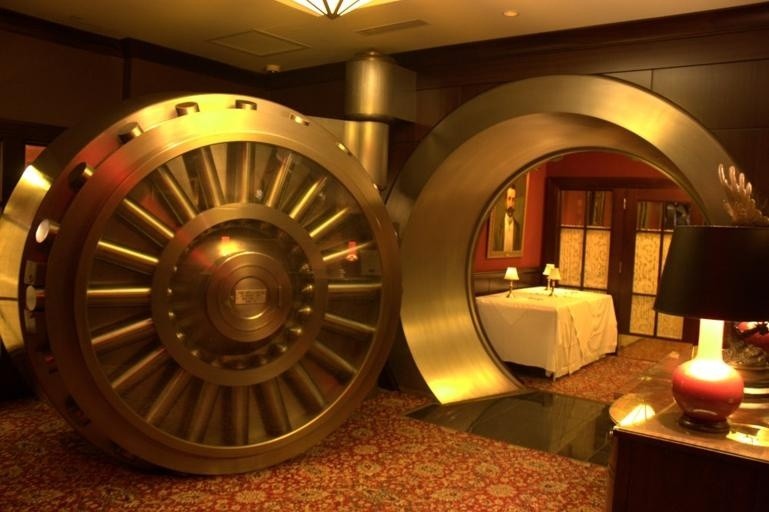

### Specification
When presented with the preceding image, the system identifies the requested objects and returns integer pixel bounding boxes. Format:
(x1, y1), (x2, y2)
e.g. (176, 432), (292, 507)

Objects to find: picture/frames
(487, 170), (530, 259)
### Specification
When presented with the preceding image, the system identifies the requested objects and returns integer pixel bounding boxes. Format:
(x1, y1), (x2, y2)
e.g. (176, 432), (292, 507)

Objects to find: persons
(493, 183), (521, 252)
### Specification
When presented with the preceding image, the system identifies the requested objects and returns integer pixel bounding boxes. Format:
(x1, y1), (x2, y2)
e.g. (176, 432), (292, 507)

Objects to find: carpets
(517, 355), (657, 403)
(1, 387), (607, 511)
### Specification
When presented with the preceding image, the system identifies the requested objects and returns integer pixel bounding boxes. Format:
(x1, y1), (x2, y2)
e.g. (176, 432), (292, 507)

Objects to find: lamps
(294, 1), (372, 20)
(652, 226), (768, 434)
(542, 264), (556, 290)
(504, 267), (519, 298)
(548, 268), (561, 297)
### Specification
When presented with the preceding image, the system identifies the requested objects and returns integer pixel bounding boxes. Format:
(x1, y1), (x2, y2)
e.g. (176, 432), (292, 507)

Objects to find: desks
(607, 341), (769, 512)
(474, 287), (618, 381)
